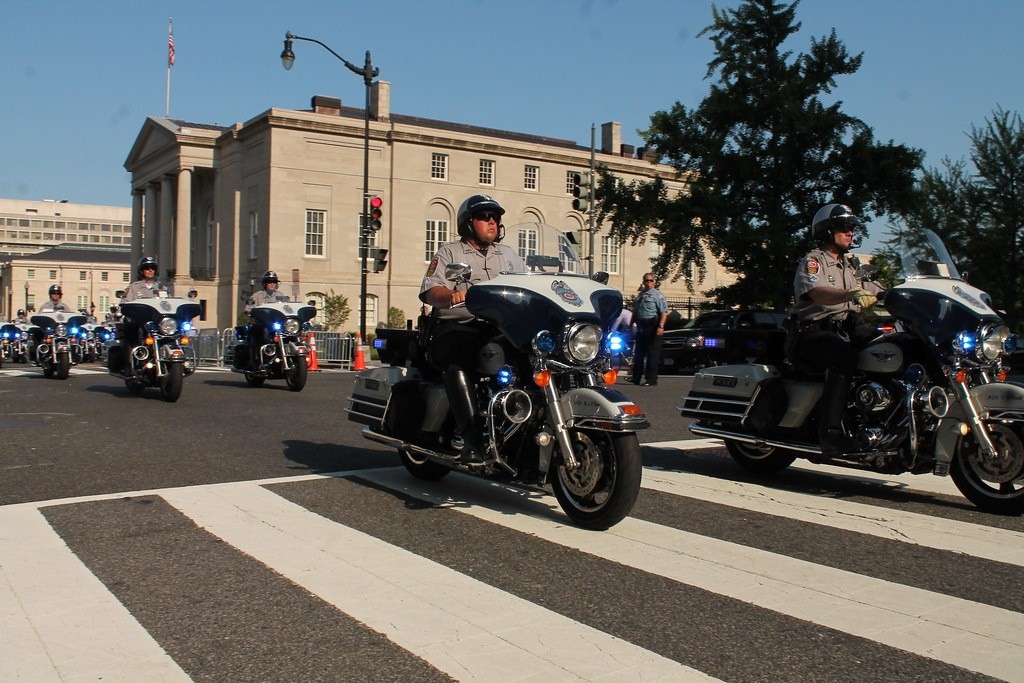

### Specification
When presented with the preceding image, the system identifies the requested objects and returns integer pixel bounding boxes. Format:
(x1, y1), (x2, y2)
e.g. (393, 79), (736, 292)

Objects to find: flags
(169, 31), (175, 65)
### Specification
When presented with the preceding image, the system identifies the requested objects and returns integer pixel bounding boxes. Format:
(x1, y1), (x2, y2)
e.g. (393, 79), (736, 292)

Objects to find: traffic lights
(368, 196), (383, 232)
(375, 246), (388, 272)
(572, 173), (588, 213)
(561, 230), (583, 260)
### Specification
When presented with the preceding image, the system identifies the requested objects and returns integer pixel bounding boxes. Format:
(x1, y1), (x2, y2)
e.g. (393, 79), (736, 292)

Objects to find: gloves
(846, 286), (877, 307)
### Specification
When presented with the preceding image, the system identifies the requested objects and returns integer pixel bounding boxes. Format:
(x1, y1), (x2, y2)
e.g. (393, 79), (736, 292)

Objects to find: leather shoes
(640, 381), (658, 386)
(624, 376), (643, 385)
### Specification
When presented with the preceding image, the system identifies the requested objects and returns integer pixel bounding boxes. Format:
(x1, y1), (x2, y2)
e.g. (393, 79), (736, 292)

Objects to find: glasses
(645, 279), (656, 282)
(144, 265), (158, 271)
(473, 210), (502, 224)
(835, 224), (861, 234)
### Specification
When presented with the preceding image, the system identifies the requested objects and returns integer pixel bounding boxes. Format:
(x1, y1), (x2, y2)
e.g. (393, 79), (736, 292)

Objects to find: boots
(443, 369), (489, 463)
(818, 371), (863, 458)
(249, 338), (262, 371)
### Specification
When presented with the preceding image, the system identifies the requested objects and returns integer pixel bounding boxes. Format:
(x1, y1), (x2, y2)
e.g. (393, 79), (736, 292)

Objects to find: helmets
(138, 256), (158, 275)
(49, 285), (63, 295)
(17, 309), (25, 316)
(456, 194), (507, 234)
(810, 204), (859, 240)
(262, 270), (282, 284)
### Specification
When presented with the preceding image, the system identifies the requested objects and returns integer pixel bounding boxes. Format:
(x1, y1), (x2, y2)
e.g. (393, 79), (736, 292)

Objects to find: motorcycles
(343, 220), (653, 530)
(0, 318), (117, 365)
(676, 224), (1023, 519)
(100, 289), (203, 402)
(228, 283), (319, 391)
(29, 297), (90, 379)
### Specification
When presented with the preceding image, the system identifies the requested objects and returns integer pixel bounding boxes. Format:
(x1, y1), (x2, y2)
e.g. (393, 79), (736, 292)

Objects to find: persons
(420, 195), (526, 450)
(420, 306), (431, 316)
(610, 309), (638, 332)
(119, 257), (169, 379)
(12, 284), (121, 351)
(625, 273), (667, 386)
(244, 271), (285, 372)
(795, 204), (878, 441)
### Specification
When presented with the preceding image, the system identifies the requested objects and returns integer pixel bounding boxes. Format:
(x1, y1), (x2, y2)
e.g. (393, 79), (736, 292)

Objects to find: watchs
(659, 325), (664, 328)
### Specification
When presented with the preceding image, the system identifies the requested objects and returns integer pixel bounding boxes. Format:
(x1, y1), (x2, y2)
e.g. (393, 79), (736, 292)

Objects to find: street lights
(278, 30), (381, 349)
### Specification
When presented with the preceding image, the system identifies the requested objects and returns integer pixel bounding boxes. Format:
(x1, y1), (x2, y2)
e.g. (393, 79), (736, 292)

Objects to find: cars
(654, 309), (762, 375)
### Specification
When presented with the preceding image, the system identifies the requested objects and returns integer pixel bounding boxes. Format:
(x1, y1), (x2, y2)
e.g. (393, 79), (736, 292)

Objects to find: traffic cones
(303, 330), (323, 373)
(349, 331), (369, 372)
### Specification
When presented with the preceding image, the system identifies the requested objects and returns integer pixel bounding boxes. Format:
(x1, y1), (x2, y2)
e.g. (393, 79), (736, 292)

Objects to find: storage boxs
(348, 364), (411, 432)
(682, 364), (789, 436)
(222, 340), (248, 368)
